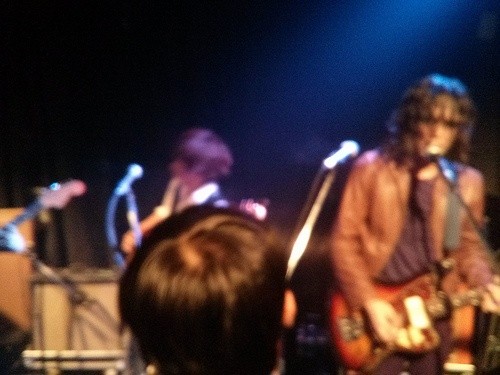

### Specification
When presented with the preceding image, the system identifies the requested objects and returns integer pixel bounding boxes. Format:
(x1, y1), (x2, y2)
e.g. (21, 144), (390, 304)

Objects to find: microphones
(31, 179), (85, 211)
(430, 147), (456, 188)
(115, 163), (142, 200)
(322, 139), (361, 171)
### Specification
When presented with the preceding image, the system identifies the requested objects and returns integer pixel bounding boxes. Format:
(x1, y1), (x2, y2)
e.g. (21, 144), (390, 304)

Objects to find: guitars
(324, 248), (499, 372)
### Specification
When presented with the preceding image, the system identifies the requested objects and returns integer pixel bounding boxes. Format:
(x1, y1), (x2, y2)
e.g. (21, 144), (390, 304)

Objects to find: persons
(119, 206), (297, 374)
(28, 166), (100, 269)
(329, 75), (486, 375)
(121, 128), (266, 374)
